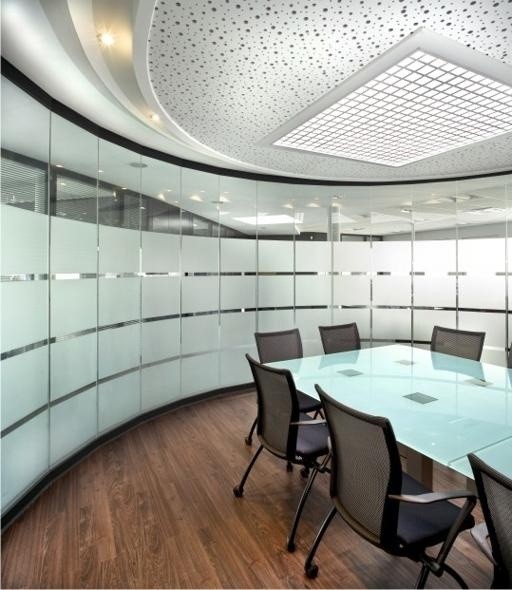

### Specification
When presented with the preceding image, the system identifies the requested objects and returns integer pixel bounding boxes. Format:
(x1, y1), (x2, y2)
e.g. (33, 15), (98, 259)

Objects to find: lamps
(253, 25), (512, 170)
